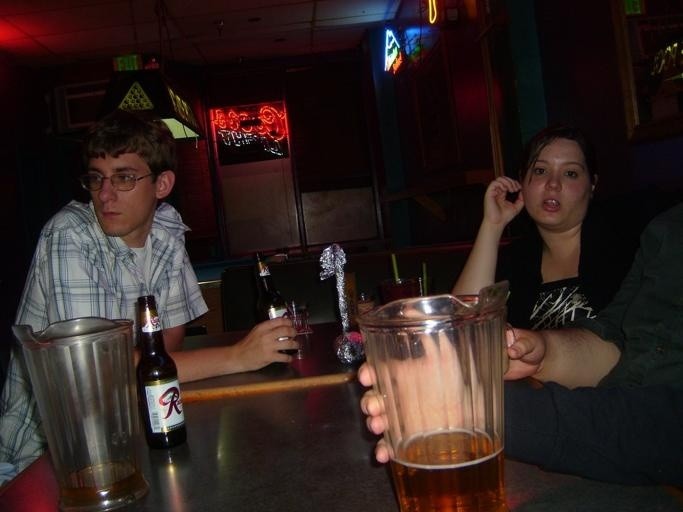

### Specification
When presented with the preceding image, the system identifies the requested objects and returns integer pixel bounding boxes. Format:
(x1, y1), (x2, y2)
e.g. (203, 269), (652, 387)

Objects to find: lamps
(95, 1), (207, 145)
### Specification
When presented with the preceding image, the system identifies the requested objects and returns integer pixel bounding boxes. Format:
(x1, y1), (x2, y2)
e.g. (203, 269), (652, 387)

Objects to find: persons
(0, 118), (301, 488)
(475, 200), (683, 390)
(356, 333), (680, 485)
(449, 126), (642, 331)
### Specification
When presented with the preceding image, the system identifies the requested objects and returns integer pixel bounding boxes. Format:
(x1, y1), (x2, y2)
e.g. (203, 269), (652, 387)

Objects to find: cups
(357, 281), (509, 512)
(12, 316), (149, 512)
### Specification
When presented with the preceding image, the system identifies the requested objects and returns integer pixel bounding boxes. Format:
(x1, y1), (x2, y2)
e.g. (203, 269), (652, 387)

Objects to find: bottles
(135, 294), (186, 450)
(250, 249), (296, 366)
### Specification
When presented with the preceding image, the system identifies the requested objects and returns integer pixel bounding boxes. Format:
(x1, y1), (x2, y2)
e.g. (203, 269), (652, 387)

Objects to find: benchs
(219, 238), (512, 333)
(230, 234), (402, 267)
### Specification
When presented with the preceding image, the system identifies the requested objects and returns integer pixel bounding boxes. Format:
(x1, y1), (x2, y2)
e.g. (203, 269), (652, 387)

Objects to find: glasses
(81, 173), (154, 192)
